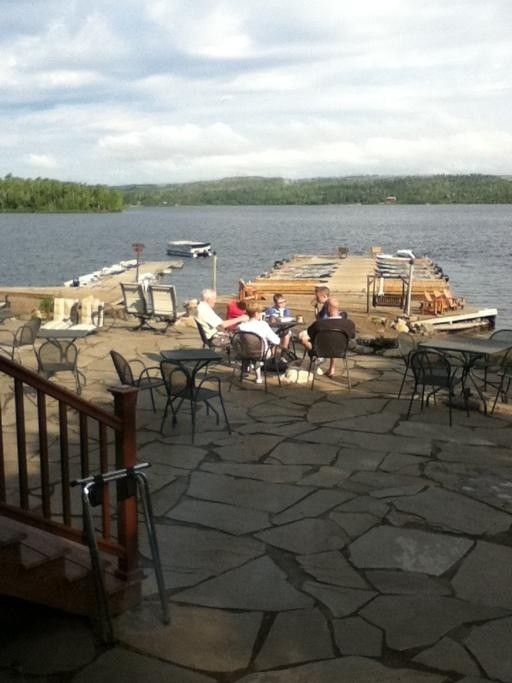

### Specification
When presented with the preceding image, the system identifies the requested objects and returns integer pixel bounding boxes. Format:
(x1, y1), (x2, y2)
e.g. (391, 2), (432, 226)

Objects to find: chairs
(395, 327), (512, 427)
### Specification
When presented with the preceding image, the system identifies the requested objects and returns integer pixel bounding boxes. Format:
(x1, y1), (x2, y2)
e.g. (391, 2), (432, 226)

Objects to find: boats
(167, 238), (216, 258)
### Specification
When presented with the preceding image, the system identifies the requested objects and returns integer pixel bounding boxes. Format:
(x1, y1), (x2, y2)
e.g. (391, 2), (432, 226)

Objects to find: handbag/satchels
(260, 348), (313, 383)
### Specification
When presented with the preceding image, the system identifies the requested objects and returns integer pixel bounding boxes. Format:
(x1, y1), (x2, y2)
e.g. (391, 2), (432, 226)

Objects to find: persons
(225, 299), (247, 328)
(237, 297), (282, 383)
(298, 284), (331, 371)
(307, 297), (353, 376)
(196, 287), (249, 348)
(262, 293), (292, 345)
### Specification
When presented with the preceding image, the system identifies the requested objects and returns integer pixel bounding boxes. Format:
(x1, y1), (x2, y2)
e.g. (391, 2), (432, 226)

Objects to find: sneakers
(256, 379), (262, 383)
(326, 368), (334, 376)
(252, 362), (264, 369)
